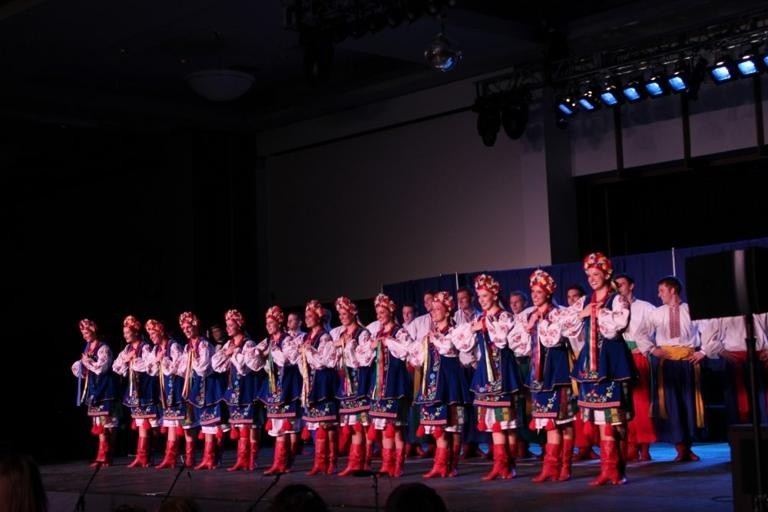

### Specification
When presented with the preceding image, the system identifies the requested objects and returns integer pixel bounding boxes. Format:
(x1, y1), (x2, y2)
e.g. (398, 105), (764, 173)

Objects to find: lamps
(417, 10), (462, 78)
(557, 18), (768, 116)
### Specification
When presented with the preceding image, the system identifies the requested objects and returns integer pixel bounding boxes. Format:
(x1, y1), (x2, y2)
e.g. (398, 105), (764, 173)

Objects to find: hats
(224, 309), (244, 326)
(374, 293), (396, 313)
(79, 318), (97, 332)
(265, 306), (284, 322)
(528, 269), (558, 294)
(582, 252), (613, 275)
(433, 291), (455, 311)
(123, 315), (141, 330)
(144, 319), (164, 332)
(335, 297), (358, 315)
(305, 300), (326, 317)
(178, 311), (200, 327)
(473, 273), (500, 294)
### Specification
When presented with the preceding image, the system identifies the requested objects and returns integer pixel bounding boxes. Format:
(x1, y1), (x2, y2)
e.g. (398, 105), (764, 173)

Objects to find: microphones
(348, 466), (390, 479)
(91, 457), (106, 467)
(267, 468), (282, 489)
(176, 453), (194, 482)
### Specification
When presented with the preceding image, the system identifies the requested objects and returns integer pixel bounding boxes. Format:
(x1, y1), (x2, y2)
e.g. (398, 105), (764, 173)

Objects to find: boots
(185, 442), (224, 470)
(263, 441), (296, 474)
(532, 440), (576, 482)
(92, 438), (110, 469)
(483, 445), (518, 481)
(128, 437), (150, 468)
(155, 440), (179, 469)
(306, 440), (470, 479)
(227, 440), (257, 472)
(591, 440), (628, 486)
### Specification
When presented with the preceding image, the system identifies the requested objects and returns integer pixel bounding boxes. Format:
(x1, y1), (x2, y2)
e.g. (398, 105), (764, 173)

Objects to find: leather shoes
(673, 445), (699, 464)
(630, 443), (651, 461)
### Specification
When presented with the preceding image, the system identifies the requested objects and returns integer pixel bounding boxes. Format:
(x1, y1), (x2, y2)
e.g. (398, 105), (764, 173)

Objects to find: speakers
(727, 423), (768, 511)
(682, 245), (768, 321)
